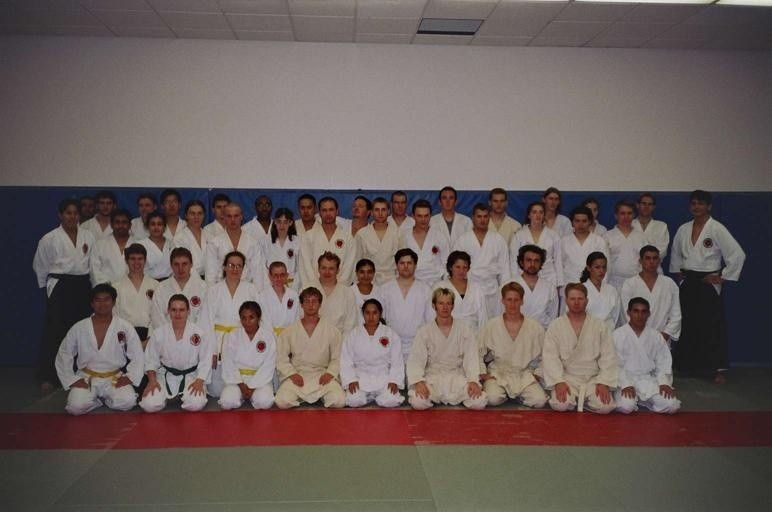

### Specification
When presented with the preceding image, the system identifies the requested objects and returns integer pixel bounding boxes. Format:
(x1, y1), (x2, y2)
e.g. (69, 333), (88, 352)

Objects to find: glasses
(227, 263), (244, 270)
